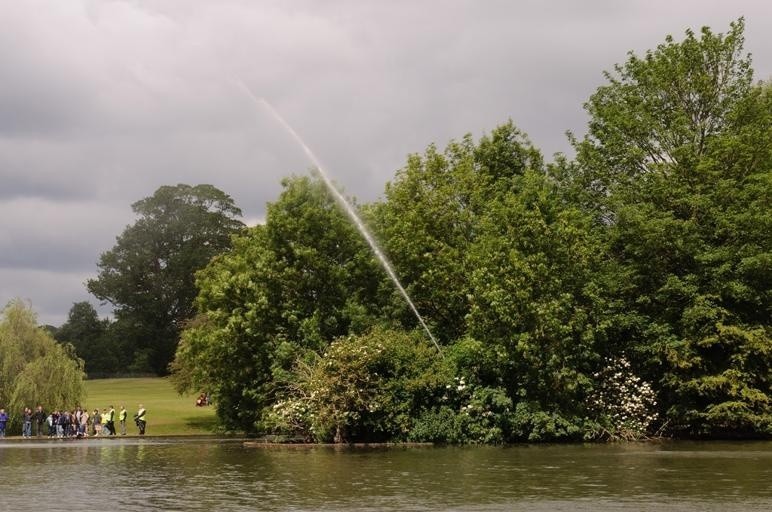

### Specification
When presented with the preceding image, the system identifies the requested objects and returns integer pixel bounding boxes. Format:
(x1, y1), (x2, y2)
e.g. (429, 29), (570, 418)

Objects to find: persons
(135, 403), (146, 435)
(22, 405), (117, 439)
(0, 408), (9, 437)
(195, 392), (212, 408)
(119, 405), (128, 435)
